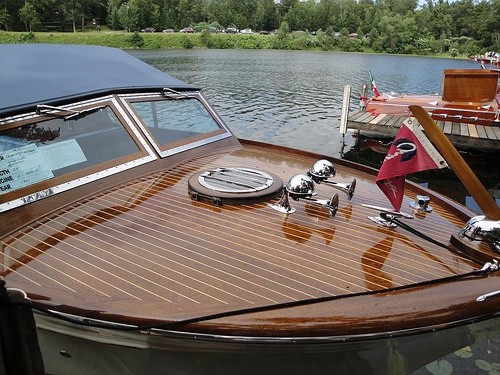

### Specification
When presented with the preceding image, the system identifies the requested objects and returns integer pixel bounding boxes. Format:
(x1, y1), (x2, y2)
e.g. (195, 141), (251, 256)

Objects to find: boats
(363, 60), (500, 127)
(1, 42), (500, 374)
